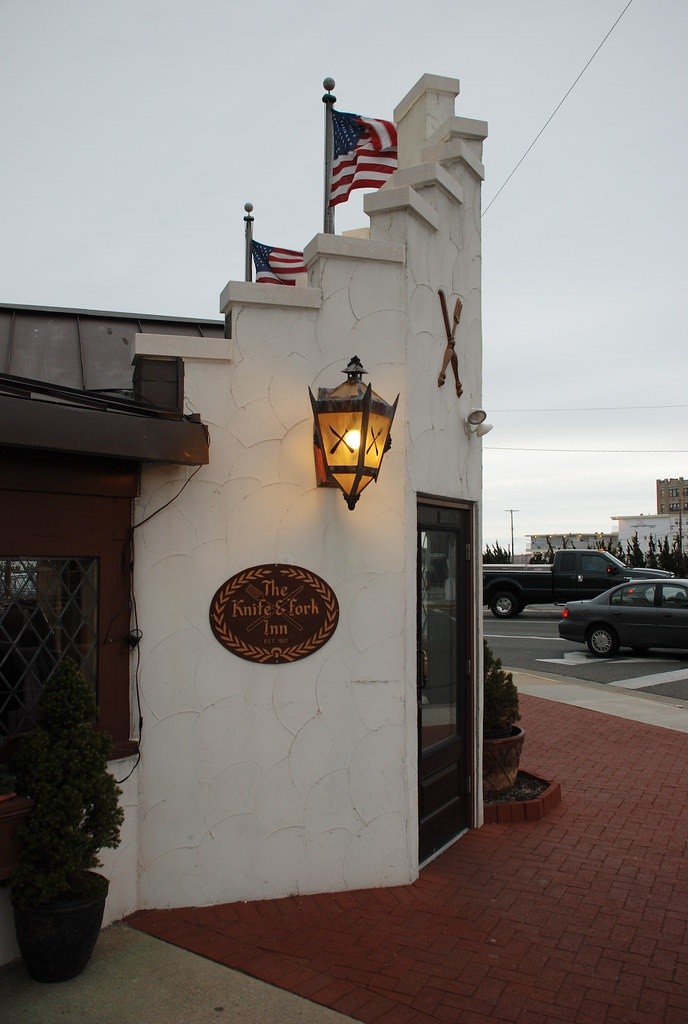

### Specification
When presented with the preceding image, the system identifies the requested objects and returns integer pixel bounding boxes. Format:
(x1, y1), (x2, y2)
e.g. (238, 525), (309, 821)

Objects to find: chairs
(677, 591), (685, 608)
(661, 595), (668, 607)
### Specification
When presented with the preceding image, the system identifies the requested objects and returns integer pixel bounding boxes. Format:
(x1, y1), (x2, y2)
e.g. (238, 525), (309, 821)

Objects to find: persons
(583, 556), (595, 570)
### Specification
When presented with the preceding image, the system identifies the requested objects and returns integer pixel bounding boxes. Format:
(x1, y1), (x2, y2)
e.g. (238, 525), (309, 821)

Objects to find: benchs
(614, 597), (652, 606)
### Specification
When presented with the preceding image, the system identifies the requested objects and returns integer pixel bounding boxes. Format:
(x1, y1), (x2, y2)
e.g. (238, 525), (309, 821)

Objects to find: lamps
(464, 410), (495, 440)
(309, 356), (401, 513)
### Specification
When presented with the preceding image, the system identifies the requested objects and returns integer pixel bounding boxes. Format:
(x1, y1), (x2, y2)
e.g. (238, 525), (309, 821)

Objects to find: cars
(557, 577), (688, 658)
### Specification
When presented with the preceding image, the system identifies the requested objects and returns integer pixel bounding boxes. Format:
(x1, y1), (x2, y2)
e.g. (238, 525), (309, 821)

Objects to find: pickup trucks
(483, 547), (675, 619)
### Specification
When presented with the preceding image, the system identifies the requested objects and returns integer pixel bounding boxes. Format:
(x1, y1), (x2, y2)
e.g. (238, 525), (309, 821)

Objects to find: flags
(246, 238), (308, 288)
(325, 109), (398, 208)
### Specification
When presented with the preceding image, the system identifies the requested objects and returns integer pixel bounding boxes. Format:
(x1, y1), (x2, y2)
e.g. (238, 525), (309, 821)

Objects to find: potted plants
(9, 665), (125, 982)
(483, 640), (527, 793)
